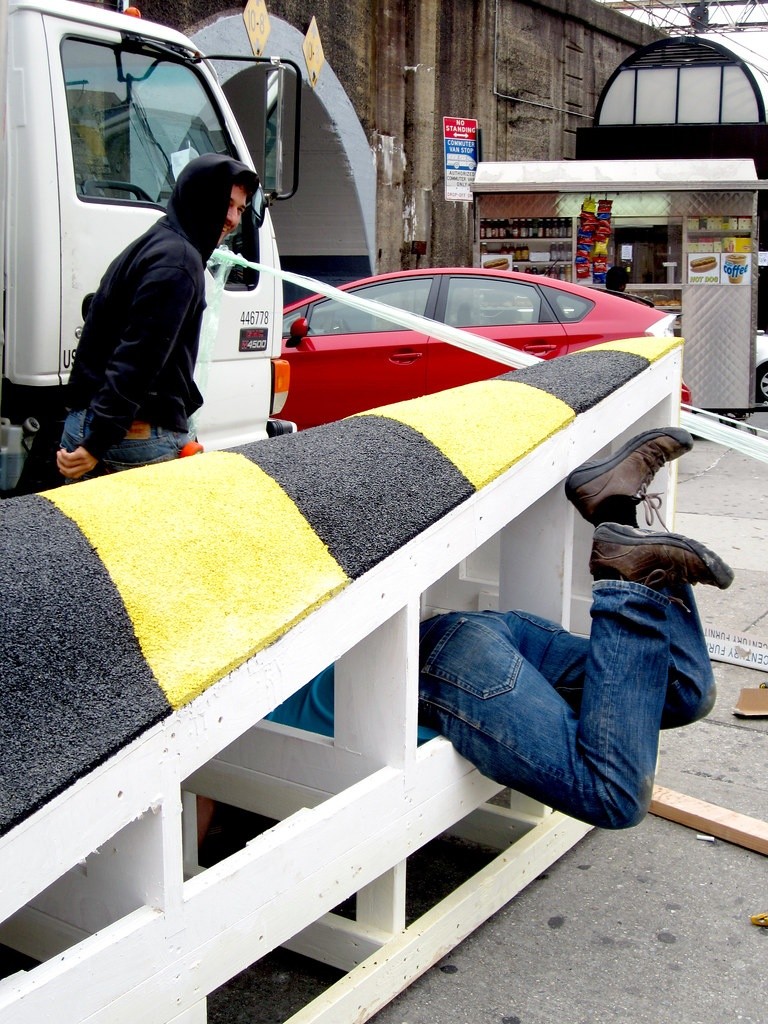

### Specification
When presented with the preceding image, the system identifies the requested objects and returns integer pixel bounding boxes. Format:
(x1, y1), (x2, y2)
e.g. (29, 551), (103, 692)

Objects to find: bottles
(480, 242), (572, 261)
(480, 218), (572, 238)
(729, 241), (734, 253)
(513, 265), (572, 283)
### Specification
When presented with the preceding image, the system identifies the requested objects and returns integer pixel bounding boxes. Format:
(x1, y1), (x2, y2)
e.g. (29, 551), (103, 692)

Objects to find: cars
(270, 267), (692, 431)
(675, 306), (768, 402)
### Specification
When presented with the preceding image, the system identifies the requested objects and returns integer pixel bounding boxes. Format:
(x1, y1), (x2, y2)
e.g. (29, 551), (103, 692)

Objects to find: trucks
(0, 1), (302, 459)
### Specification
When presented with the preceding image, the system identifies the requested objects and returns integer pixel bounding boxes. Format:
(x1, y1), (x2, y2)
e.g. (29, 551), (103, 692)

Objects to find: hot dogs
(483, 258), (509, 270)
(690, 256), (717, 272)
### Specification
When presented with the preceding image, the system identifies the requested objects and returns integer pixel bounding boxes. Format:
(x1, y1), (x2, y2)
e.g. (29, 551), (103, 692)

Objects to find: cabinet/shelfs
(594, 284), (682, 330)
(473, 213), (577, 285)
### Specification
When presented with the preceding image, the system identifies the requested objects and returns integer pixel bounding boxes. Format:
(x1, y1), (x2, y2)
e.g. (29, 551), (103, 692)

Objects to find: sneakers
(588, 521), (735, 591)
(563, 426), (694, 533)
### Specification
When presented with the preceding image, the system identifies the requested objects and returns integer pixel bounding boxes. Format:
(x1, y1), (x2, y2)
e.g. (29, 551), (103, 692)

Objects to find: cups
(725, 254), (747, 284)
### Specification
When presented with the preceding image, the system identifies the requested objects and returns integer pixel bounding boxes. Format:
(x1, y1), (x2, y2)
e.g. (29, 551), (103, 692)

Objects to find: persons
(57, 153), (261, 487)
(196, 425), (733, 841)
